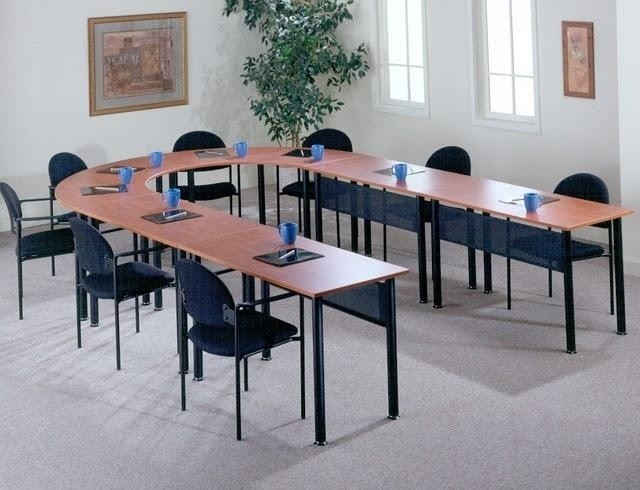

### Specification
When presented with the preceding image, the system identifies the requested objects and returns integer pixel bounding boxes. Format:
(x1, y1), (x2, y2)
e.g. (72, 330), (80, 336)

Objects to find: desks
(55, 143), (635, 445)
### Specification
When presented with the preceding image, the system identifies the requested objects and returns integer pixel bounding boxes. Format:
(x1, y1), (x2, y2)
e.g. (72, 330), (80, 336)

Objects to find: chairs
(278, 127), (357, 252)
(164, 127), (240, 219)
(46, 149), (129, 236)
(506, 171), (616, 315)
(175, 258), (306, 443)
(70, 219), (177, 372)
(0, 180), (88, 324)
(382, 145), (472, 281)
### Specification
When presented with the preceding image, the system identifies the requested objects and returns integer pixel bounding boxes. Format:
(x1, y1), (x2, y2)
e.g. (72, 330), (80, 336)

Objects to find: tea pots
(524, 193), (544, 212)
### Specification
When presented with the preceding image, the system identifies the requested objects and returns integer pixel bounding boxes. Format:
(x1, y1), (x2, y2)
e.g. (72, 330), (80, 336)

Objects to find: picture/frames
(556, 19), (598, 100)
(84, 9), (189, 118)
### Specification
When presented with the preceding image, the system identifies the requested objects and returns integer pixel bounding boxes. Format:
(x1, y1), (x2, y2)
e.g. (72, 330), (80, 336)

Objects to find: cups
(278, 222), (296, 244)
(273, 244), (297, 262)
(391, 164), (408, 182)
(161, 187), (180, 210)
(147, 152), (163, 168)
(232, 143), (247, 158)
(311, 144), (324, 160)
(116, 167), (133, 186)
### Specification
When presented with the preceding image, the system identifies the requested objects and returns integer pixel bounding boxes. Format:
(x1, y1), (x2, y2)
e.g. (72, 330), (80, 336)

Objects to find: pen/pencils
(512, 199), (526, 201)
(279, 250), (295, 260)
(163, 212), (187, 220)
(409, 166), (414, 173)
(94, 187), (120, 191)
(300, 149), (304, 157)
(110, 168), (137, 173)
(206, 152), (223, 156)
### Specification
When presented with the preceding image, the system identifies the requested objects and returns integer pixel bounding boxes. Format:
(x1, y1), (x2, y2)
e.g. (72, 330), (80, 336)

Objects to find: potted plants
(217, 0), (376, 196)
